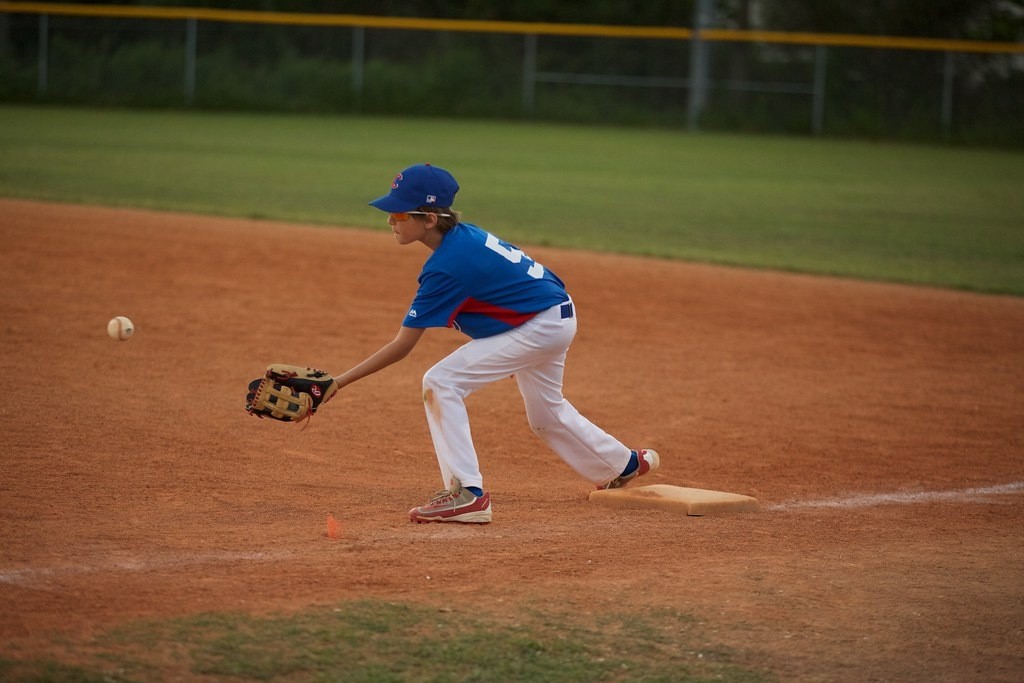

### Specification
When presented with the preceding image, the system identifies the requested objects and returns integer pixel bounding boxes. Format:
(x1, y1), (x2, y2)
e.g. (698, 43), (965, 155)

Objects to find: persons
(247, 162), (659, 524)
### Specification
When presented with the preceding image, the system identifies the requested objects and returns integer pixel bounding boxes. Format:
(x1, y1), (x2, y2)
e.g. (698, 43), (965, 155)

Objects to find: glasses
(388, 210), (452, 222)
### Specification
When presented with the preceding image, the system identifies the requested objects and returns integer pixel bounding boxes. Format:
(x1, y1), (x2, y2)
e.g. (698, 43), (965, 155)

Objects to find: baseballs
(106, 316), (135, 340)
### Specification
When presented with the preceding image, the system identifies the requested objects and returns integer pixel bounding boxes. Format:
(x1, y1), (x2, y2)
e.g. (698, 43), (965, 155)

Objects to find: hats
(369, 161), (461, 213)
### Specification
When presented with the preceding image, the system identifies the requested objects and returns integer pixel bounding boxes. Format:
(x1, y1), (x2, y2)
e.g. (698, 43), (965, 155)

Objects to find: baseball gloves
(245, 363), (338, 432)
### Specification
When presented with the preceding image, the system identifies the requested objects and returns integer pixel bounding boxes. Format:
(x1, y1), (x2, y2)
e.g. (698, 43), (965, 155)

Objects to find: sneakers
(597, 449), (660, 491)
(409, 475), (492, 524)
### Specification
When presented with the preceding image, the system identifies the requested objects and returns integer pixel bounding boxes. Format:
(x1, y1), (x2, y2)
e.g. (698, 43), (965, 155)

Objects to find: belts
(560, 302), (574, 319)
